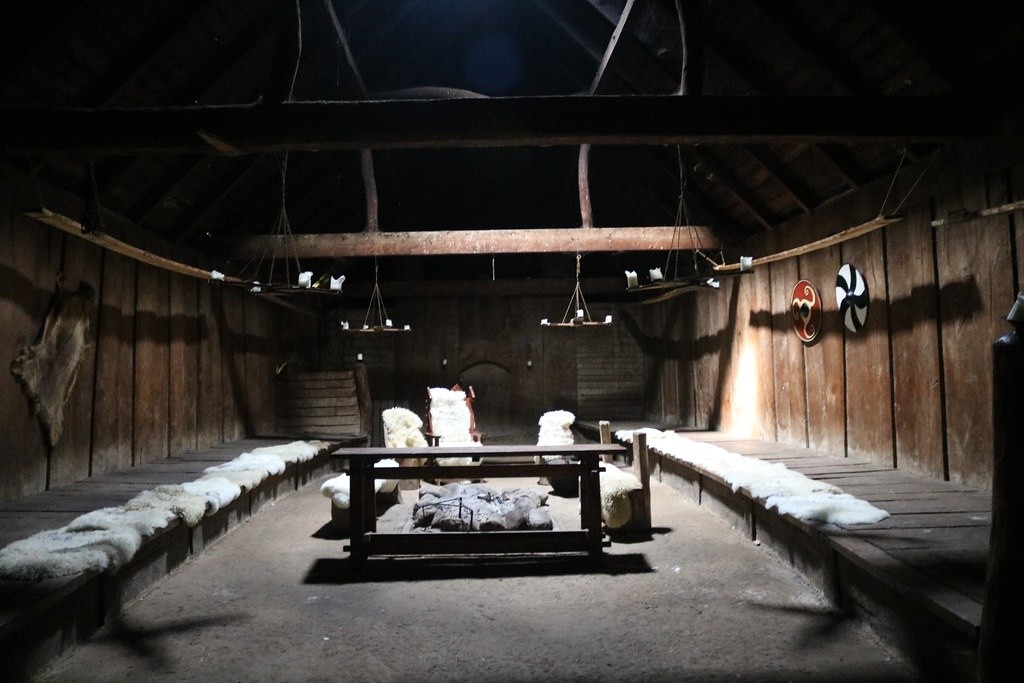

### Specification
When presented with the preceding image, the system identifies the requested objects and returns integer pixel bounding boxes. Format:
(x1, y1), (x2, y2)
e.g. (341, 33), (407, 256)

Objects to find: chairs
(427, 387), (482, 477)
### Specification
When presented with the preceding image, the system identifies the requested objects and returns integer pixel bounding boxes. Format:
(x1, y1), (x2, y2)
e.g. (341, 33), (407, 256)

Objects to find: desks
(330, 443), (629, 566)
